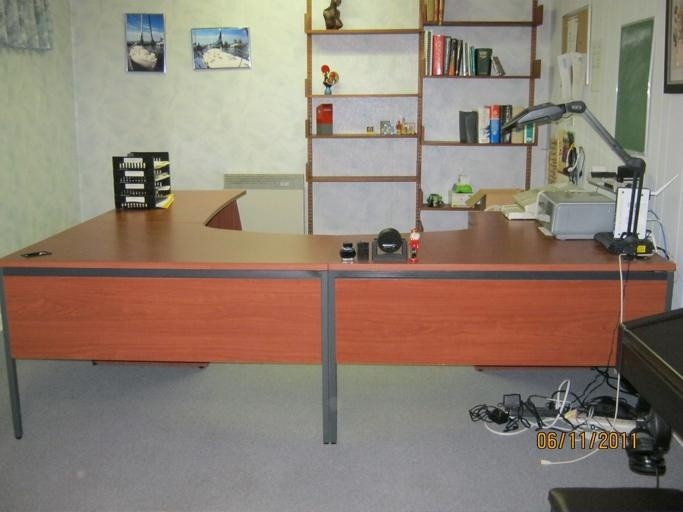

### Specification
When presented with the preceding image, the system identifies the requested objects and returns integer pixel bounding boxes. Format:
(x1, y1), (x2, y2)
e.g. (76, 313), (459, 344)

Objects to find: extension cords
(508, 406), (567, 417)
(569, 413), (645, 433)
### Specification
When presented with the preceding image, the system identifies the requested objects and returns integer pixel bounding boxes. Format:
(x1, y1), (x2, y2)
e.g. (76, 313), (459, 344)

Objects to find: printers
(535, 191), (617, 240)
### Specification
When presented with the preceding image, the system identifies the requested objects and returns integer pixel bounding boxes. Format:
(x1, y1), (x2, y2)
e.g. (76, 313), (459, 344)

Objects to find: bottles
(339, 241), (356, 260)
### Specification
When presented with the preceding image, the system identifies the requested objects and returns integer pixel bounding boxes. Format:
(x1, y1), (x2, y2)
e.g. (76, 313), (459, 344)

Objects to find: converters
(503, 394), (520, 408)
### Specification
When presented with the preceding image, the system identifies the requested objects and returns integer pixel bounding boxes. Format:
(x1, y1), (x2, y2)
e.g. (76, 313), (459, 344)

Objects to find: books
(424, 30), (507, 77)
(459, 107), (535, 144)
(423, 0), (444, 23)
(119, 157), (175, 209)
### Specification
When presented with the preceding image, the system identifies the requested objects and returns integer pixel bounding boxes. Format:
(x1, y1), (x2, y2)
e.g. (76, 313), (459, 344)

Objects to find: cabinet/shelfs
(298, 1), (545, 236)
(111, 149), (172, 211)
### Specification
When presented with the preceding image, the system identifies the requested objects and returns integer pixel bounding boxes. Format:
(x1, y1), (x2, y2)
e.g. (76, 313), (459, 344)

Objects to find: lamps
(502, 95), (655, 259)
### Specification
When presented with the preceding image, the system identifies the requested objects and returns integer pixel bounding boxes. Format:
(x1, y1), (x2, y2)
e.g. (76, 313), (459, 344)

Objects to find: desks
(0, 188), (677, 451)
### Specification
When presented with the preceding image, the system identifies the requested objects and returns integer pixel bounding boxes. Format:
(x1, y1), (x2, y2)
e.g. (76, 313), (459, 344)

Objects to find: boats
(129, 45), (158, 69)
(201, 47), (251, 68)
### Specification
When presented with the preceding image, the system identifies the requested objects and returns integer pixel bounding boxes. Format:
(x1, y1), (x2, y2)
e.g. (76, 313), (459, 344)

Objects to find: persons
(321, 65), (339, 94)
(408, 227), (422, 262)
